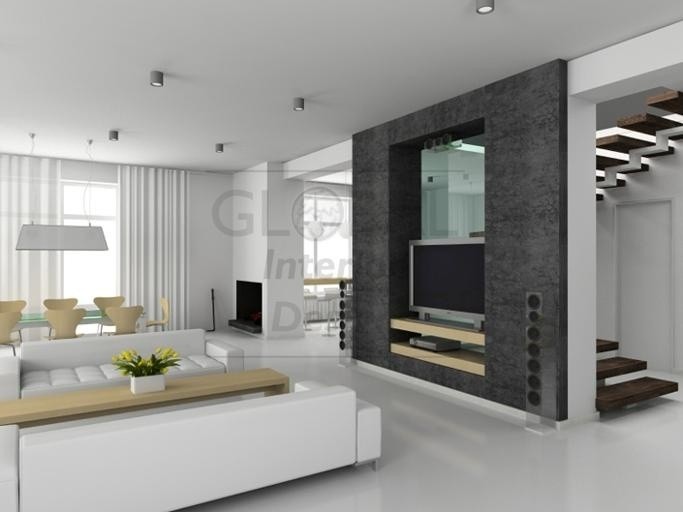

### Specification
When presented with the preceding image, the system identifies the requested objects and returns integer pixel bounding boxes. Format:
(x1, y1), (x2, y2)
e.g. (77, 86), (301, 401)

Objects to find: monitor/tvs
(406, 236), (484, 333)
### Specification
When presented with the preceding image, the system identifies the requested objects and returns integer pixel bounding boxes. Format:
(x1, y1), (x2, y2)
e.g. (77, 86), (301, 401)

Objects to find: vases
(131, 375), (165, 394)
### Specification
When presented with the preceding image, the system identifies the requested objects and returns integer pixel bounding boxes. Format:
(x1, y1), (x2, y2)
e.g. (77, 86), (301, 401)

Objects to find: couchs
(0, 328), (245, 409)
(0, 380), (382, 512)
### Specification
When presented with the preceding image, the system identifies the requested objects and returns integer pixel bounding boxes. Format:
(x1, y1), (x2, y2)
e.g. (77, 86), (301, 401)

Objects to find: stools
(302, 288), (339, 337)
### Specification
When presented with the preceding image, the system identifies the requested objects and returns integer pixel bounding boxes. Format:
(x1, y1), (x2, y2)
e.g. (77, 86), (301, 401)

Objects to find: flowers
(111, 348), (180, 377)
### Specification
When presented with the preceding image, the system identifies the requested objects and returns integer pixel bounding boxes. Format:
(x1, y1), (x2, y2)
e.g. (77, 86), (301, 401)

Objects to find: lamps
(16, 133), (109, 252)
(295, 96), (305, 112)
(475, 1), (494, 15)
(149, 71), (165, 87)
(216, 144), (223, 153)
(109, 131), (119, 141)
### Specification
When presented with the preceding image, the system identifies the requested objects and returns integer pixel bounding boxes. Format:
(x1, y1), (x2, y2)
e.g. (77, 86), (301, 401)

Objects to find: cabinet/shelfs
(385, 318), (485, 376)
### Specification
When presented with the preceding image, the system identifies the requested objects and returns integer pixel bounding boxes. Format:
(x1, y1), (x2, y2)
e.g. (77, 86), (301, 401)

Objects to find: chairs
(0, 297), (169, 357)
